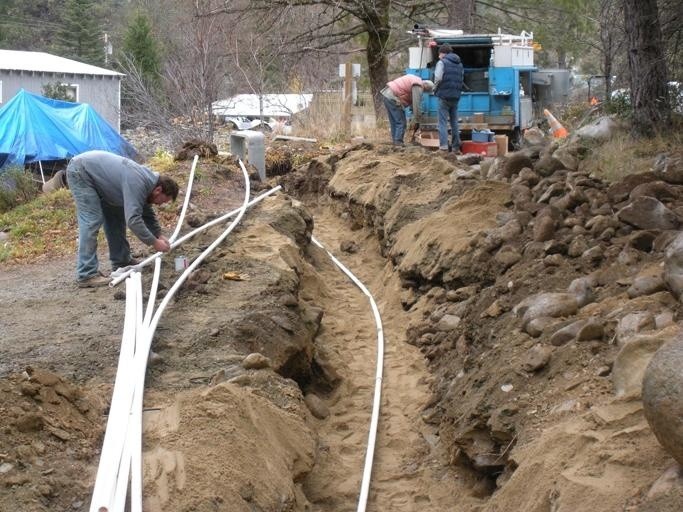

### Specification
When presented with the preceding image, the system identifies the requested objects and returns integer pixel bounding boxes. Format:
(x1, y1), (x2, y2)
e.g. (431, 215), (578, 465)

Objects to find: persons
(434, 41), (465, 154)
(380, 74), (435, 148)
(65, 149), (179, 288)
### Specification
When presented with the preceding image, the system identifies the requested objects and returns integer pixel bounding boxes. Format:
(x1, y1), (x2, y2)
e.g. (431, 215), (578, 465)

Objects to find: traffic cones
(541, 109), (569, 139)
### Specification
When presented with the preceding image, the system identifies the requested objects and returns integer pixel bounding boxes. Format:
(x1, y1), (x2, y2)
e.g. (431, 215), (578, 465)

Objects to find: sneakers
(128, 257), (143, 265)
(78, 274), (111, 288)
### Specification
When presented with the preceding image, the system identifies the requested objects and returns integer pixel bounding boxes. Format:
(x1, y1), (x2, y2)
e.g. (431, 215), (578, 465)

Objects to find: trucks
(612, 82), (683, 115)
(403, 24), (569, 152)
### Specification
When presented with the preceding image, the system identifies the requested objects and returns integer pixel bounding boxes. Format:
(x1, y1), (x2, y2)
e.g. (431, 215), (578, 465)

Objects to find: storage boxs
(414, 129), (508, 156)
